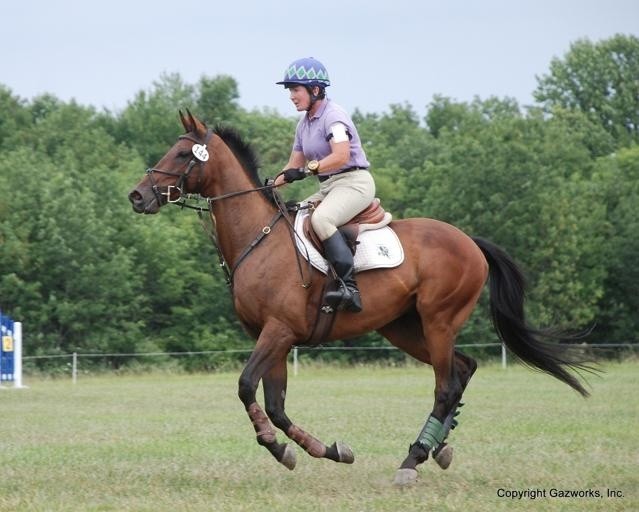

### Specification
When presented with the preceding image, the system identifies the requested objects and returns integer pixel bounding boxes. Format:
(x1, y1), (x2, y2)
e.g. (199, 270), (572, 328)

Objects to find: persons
(264, 59), (377, 313)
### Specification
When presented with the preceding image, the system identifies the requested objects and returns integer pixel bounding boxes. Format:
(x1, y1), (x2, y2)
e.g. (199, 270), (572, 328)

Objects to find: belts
(318, 166), (366, 183)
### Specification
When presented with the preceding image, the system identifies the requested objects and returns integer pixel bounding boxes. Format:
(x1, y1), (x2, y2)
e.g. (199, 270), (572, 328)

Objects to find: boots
(321, 230), (362, 313)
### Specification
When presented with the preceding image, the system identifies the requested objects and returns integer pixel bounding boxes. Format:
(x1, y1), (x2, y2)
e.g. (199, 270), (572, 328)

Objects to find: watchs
(306, 157), (321, 177)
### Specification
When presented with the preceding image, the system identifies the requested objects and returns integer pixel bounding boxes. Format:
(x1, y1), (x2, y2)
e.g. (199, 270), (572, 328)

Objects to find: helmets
(276, 57), (330, 88)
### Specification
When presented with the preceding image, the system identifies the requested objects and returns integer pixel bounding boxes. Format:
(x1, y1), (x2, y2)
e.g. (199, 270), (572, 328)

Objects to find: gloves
(283, 168), (306, 183)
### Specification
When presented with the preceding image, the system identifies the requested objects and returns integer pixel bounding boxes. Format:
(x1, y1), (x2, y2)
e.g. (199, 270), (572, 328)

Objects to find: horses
(126, 106), (606, 487)
